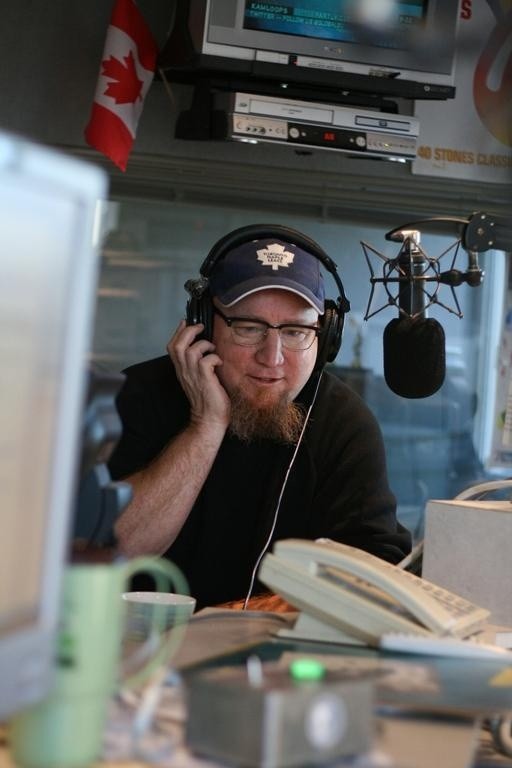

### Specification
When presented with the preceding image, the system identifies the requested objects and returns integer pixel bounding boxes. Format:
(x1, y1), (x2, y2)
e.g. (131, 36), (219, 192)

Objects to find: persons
(106, 222), (414, 615)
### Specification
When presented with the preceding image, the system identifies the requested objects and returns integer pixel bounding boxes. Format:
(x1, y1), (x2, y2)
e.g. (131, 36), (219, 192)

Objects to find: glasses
(213, 305), (325, 354)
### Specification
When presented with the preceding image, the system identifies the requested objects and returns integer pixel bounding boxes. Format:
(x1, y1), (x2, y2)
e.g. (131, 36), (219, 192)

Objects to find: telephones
(257, 537), (491, 649)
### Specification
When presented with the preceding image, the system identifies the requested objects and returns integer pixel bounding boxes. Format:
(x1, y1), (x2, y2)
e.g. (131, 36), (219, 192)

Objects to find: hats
(212, 239), (329, 316)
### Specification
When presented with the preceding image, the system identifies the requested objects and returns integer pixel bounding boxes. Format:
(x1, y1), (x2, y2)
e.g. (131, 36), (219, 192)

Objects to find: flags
(81, 0), (161, 173)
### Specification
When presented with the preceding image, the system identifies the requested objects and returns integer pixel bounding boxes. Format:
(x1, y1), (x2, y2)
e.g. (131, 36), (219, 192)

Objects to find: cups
(6, 558), (188, 768)
(122, 590), (196, 654)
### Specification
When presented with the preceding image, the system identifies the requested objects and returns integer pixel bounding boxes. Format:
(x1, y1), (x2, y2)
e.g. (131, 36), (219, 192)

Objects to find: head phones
(184, 224), (351, 376)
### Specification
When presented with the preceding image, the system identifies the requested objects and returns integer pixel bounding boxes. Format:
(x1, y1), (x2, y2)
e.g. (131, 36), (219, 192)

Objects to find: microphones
(384, 231), (446, 399)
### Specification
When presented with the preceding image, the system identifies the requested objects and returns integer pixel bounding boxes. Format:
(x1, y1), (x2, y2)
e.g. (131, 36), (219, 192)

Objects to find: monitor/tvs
(200, 0), (462, 116)
